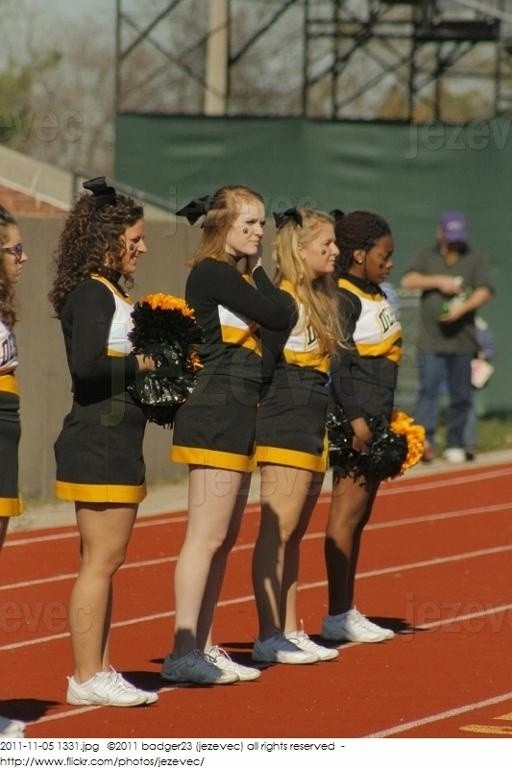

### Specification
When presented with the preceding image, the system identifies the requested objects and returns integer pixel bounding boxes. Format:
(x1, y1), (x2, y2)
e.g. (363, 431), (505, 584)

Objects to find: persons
(461, 315), (496, 461)
(251, 207), (340, 665)
(399, 210), (497, 466)
(160, 184), (298, 685)
(0, 206), (29, 738)
(48, 176), (159, 708)
(320, 209), (395, 643)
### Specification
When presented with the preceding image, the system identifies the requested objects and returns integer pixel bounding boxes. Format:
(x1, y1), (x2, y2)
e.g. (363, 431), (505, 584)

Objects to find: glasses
(2, 246), (22, 261)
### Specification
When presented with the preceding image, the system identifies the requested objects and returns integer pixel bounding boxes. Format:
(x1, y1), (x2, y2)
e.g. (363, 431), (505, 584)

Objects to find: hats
(442, 213), (466, 242)
